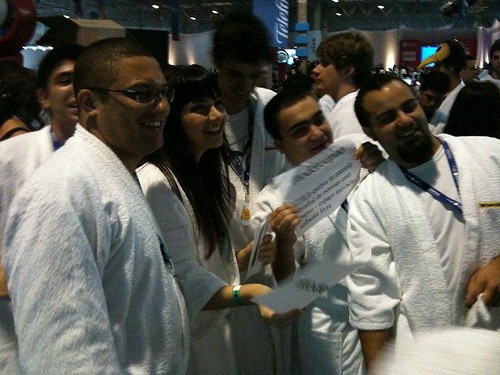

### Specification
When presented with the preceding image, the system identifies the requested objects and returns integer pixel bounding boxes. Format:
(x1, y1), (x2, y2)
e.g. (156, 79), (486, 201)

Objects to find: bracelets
(231, 284), (246, 307)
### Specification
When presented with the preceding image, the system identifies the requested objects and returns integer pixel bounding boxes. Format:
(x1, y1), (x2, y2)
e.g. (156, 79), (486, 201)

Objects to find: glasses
(88, 86), (175, 103)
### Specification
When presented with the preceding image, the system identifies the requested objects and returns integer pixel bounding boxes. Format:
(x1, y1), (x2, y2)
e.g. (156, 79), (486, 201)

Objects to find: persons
(0, 43), (89, 375)
(195, 12), (304, 375)
(0, 36), (189, 375)
(249, 84), (384, 375)
(345, 70), (500, 375)
(273, 29), (500, 160)
(135, 62), (278, 375)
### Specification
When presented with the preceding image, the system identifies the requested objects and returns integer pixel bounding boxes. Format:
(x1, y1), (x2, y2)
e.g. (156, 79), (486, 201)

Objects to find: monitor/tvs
(420, 45), (439, 68)
(284, 49), (296, 65)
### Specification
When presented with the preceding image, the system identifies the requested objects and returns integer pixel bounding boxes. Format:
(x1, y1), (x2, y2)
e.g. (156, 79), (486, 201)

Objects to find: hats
(416, 42), (465, 71)
